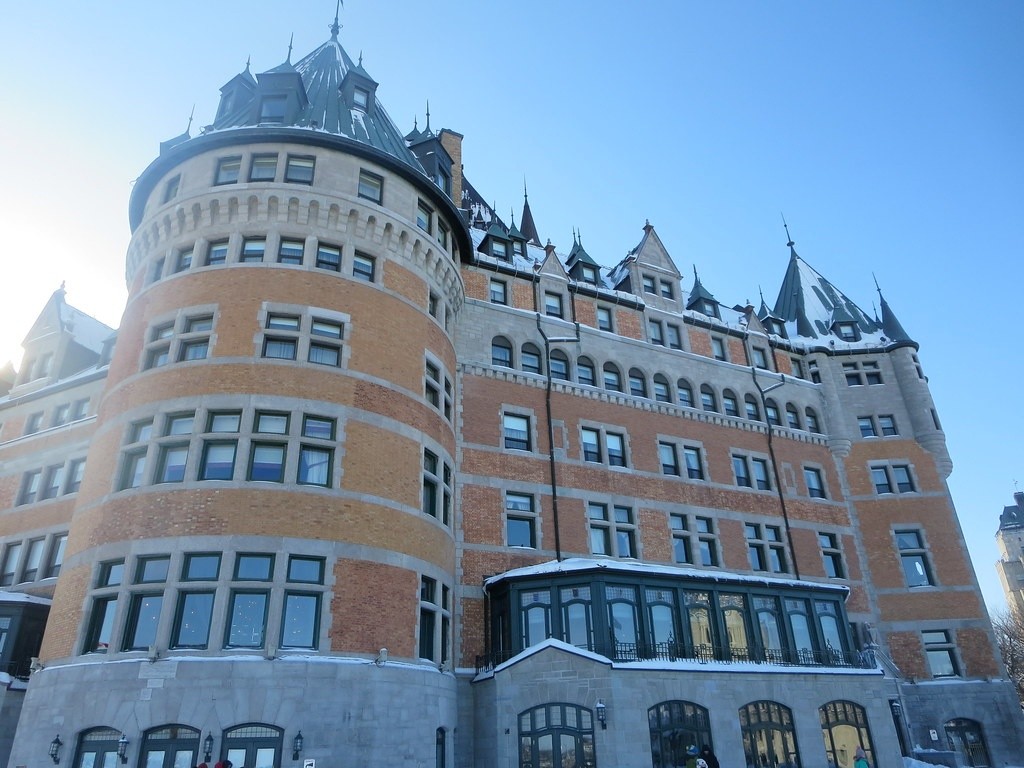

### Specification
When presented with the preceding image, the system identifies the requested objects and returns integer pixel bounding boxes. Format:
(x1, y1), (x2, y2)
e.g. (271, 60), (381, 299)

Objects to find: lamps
(117, 734), (130, 764)
(595, 700), (608, 730)
(292, 730), (304, 761)
(891, 701), (902, 718)
(48, 733), (63, 764)
(203, 731), (214, 762)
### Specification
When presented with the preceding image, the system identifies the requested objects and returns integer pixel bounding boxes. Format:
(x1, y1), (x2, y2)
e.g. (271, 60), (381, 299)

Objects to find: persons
(686, 745), (719, 768)
(198, 760), (233, 768)
(746, 748), (779, 768)
(853, 747), (868, 768)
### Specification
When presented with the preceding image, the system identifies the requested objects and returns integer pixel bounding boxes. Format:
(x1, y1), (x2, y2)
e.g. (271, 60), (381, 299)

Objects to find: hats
(855, 749), (865, 761)
